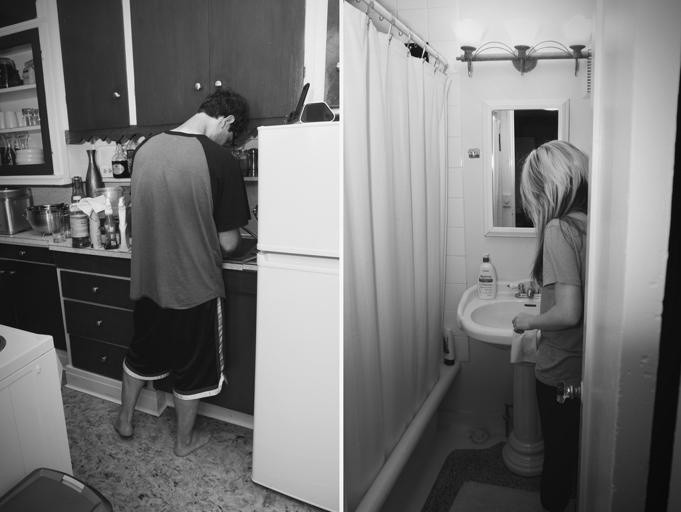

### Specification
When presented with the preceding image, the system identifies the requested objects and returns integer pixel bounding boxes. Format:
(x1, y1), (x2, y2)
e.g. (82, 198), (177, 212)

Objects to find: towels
(509, 329), (539, 367)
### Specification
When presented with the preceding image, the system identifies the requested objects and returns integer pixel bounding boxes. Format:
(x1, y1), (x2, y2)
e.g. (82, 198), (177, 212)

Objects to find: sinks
(221, 235), (257, 263)
(458, 282), (542, 337)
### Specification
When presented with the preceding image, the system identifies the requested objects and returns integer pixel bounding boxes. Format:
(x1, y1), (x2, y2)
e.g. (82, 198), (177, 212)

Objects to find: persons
(112, 88), (251, 457)
(512, 138), (589, 512)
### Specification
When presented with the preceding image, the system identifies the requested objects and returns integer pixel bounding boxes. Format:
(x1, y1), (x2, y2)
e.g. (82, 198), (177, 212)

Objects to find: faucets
(240, 226), (257, 240)
(527, 289), (534, 297)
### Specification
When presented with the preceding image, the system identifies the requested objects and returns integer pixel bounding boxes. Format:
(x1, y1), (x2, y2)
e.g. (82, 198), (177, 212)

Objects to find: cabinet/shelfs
(49, 246), (158, 419)
(56, 0), (130, 130)
(0, 27), (55, 175)
(0, 243), (68, 352)
(131, 0), (305, 127)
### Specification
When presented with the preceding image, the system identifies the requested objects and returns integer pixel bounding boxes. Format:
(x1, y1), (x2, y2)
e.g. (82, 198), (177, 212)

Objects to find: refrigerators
(250, 120), (342, 512)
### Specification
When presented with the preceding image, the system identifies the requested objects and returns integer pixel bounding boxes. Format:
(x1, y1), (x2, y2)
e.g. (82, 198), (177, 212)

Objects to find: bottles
(111, 141), (128, 178)
(69, 176), (90, 248)
(0, 133), (15, 166)
(105, 197), (117, 248)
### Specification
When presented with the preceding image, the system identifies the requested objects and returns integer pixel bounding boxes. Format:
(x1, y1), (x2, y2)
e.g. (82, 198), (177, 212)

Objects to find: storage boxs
(0, 467), (113, 512)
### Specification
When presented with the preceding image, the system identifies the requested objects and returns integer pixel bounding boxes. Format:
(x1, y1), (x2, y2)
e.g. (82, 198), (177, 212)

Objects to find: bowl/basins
(15, 148), (44, 165)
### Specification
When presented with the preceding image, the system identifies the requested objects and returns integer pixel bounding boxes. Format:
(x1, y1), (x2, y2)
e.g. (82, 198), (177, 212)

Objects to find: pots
(21, 203), (69, 235)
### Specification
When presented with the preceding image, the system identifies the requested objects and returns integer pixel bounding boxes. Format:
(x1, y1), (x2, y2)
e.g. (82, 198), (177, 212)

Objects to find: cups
(0, 107), (41, 130)
